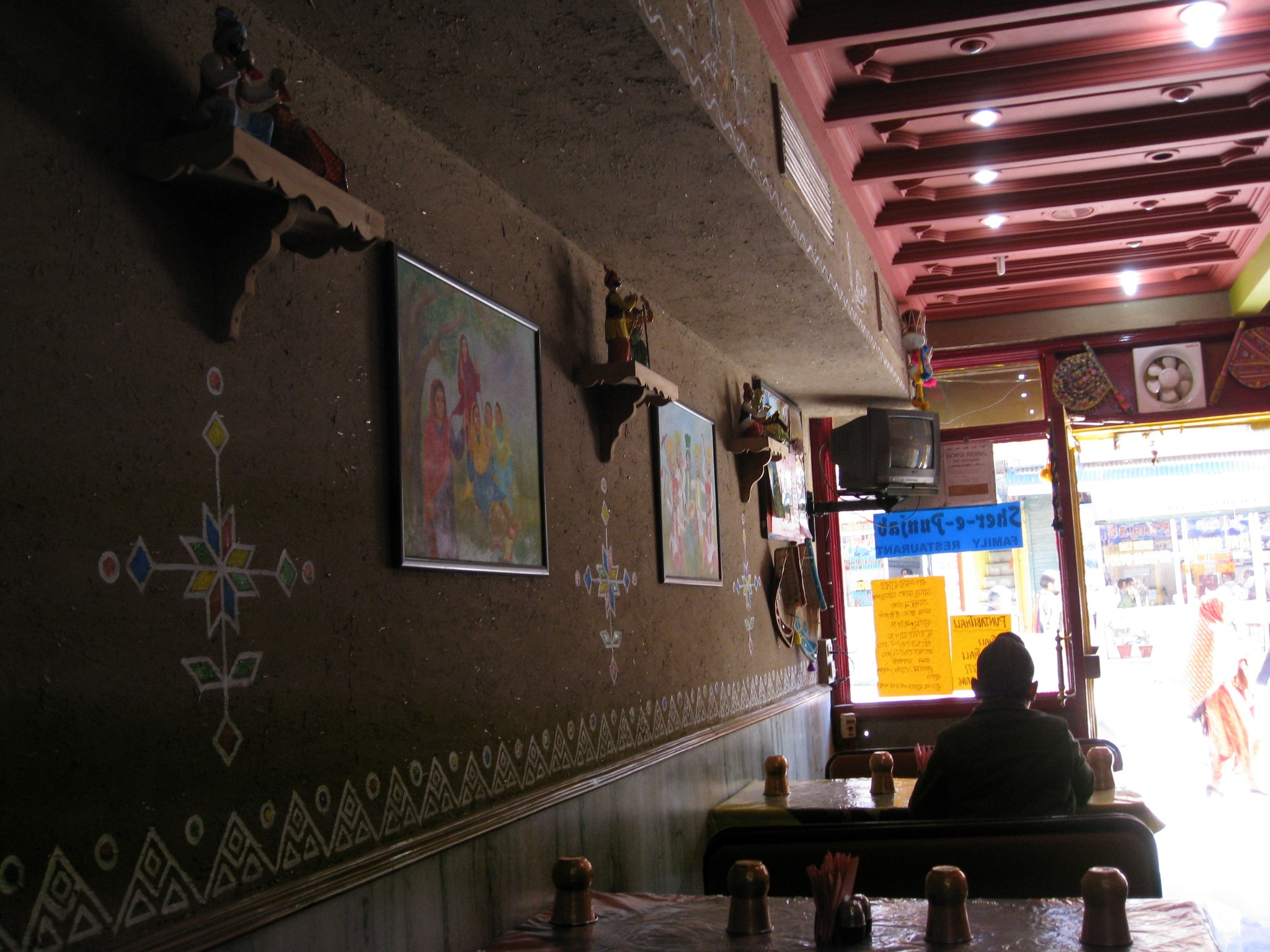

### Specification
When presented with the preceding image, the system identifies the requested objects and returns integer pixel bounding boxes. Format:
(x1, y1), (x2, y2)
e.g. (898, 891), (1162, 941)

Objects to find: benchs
(701, 814), (1163, 900)
(823, 739), (1123, 774)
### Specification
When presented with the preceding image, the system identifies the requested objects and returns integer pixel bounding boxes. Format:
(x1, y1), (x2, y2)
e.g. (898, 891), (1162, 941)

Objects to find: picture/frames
(653, 397), (724, 591)
(753, 378), (808, 545)
(380, 240), (552, 579)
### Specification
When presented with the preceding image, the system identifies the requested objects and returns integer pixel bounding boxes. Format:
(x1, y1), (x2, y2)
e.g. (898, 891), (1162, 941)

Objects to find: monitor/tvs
(830, 407), (940, 495)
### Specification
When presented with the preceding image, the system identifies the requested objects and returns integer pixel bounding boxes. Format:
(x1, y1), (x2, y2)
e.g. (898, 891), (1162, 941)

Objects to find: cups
(763, 755), (791, 796)
(549, 856), (598, 926)
(869, 751), (896, 793)
(923, 865), (973, 945)
(1078, 867), (1133, 946)
(1086, 746), (1115, 790)
(725, 861), (774, 937)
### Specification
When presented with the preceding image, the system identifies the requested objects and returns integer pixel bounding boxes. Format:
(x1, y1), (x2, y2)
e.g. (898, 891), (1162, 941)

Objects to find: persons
(898, 569), (1270, 796)
(908, 631), (1097, 819)
(424, 334), (523, 565)
(667, 431), (722, 580)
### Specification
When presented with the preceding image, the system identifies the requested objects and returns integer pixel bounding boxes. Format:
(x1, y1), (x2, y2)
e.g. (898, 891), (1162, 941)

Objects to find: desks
(488, 898), (1216, 951)
(710, 778), (1167, 836)
